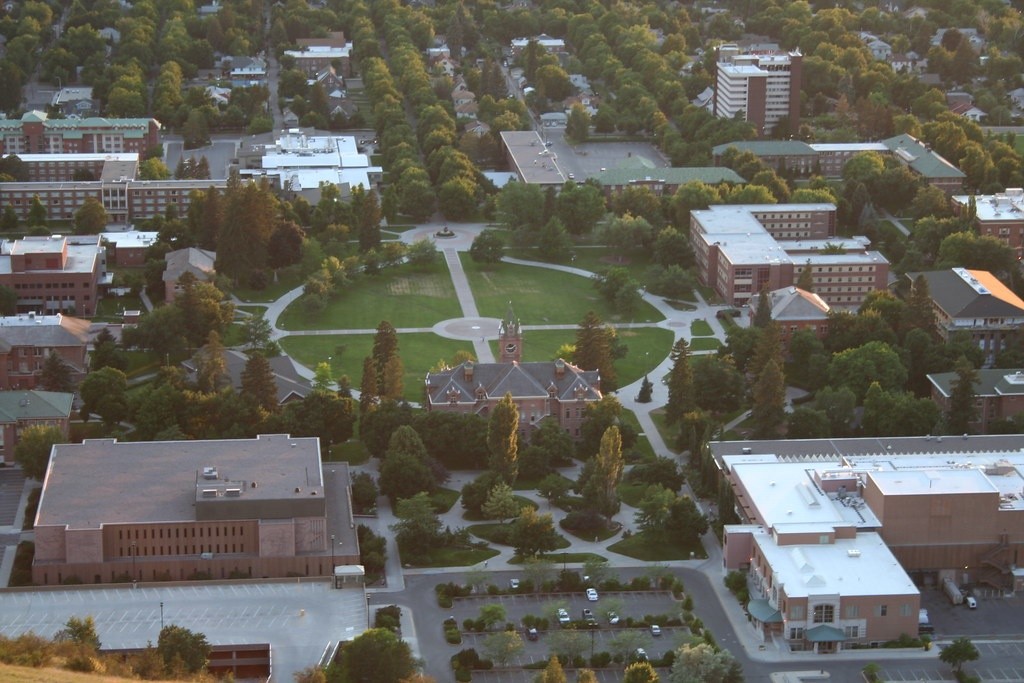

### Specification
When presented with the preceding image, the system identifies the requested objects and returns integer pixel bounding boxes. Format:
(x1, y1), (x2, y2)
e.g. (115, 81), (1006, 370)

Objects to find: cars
(557, 608), (570, 625)
(568, 173), (574, 179)
(510, 579), (519, 589)
(528, 627), (538, 641)
(634, 648), (649, 662)
(651, 625), (660, 635)
(544, 141), (553, 147)
(587, 588), (598, 601)
(607, 612), (619, 624)
(600, 167), (606, 174)
(583, 609), (598, 627)
